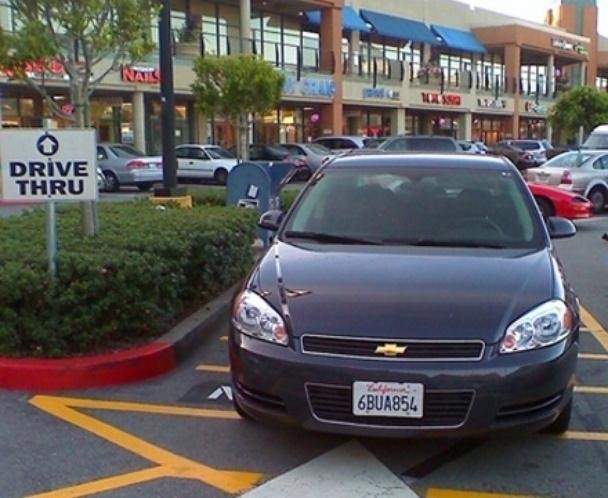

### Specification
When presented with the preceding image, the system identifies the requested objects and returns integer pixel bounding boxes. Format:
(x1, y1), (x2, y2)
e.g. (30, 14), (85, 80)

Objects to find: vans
(582, 123), (608, 149)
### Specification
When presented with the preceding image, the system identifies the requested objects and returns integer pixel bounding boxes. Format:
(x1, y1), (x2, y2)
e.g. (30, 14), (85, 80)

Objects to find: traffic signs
(0, 127), (98, 204)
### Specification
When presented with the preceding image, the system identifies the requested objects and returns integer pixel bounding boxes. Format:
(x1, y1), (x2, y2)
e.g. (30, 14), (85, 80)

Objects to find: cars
(174, 143), (242, 184)
(363, 135), (488, 154)
(524, 150), (608, 222)
(498, 138), (554, 167)
(229, 147), (579, 444)
(231, 136), (377, 181)
(97, 143), (163, 192)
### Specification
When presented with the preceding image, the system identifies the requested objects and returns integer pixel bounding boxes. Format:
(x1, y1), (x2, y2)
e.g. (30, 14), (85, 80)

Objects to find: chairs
(396, 182), (446, 214)
(454, 187), (496, 222)
(350, 184), (396, 238)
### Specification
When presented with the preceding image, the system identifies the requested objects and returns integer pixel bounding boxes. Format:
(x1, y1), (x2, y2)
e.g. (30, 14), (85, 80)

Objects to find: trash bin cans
(223, 160), (307, 247)
